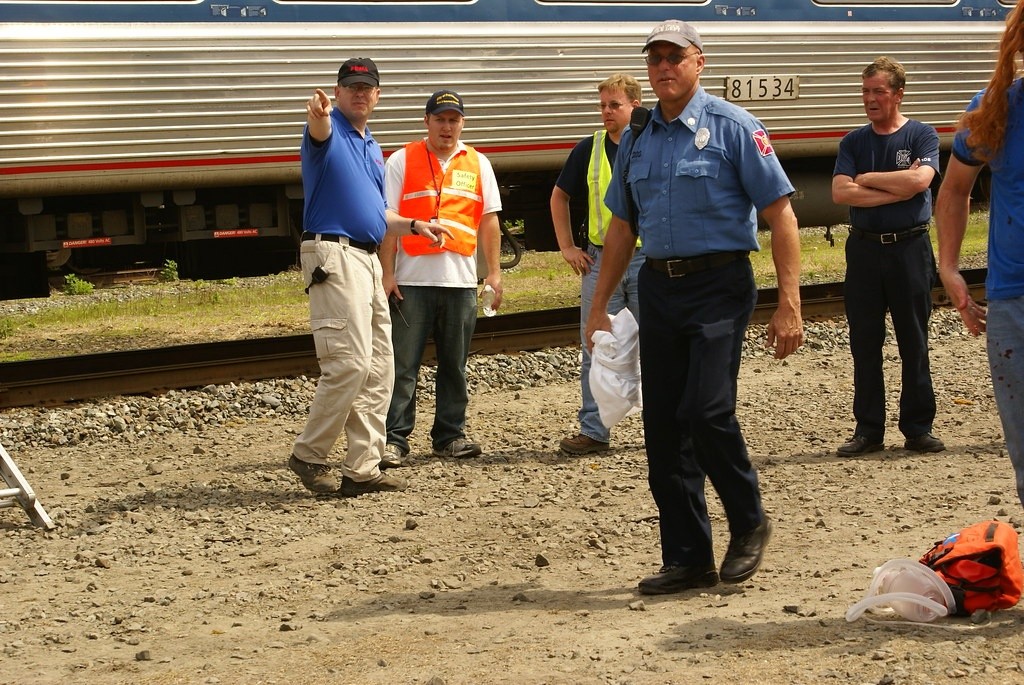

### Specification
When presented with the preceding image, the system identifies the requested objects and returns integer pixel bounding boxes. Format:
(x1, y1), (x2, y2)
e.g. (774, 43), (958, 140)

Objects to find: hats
(337, 57), (379, 88)
(642, 19), (703, 55)
(426, 90), (464, 117)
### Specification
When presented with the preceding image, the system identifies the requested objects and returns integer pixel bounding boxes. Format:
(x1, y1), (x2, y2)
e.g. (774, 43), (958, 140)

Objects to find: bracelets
(410, 219), (420, 235)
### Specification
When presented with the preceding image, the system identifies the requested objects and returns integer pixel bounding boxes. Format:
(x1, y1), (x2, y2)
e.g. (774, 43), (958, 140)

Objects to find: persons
(830, 55), (945, 456)
(584, 20), (806, 595)
(550, 75), (647, 455)
(286, 57), (453, 496)
(384, 90), (504, 467)
(934, 0), (1024, 512)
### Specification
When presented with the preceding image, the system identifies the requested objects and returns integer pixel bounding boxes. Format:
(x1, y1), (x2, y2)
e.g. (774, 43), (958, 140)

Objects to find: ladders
(0, 443), (57, 532)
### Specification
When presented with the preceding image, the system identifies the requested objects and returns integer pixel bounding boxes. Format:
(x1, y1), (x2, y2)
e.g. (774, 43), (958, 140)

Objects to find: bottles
(873, 567), (945, 624)
(482, 285), (496, 317)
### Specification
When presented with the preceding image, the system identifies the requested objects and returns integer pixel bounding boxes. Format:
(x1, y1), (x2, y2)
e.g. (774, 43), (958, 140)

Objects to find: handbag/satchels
(919, 521), (1024, 619)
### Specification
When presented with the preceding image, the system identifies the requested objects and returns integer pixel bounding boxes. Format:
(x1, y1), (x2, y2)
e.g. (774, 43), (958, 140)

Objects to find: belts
(849, 225), (929, 244)
(645, 250), (751, 277)
(300, 232), (378, 254)
(589, 241), (641, 251)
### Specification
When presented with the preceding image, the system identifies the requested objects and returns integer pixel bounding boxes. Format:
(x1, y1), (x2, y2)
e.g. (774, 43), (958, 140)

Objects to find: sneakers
(379, 444), (408, 466)
(559, 434), (609, 454)
(341, 471), (407, 494)
(719, 511), (772, 582)
(905, 431), (946, 453)
(835, 423), (885, 457)
(433, 437), (482, 458)
(638, 559), (719, 594)
(288, 454), (336, 494)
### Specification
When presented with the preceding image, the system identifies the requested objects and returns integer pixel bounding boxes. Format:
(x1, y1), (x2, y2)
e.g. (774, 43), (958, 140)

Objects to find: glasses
(644, 52), (701, 65)
(339, 83), (378, 94)
(594, 99), (634, 112)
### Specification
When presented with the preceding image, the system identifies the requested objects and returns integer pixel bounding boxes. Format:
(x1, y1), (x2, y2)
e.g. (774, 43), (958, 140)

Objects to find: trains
(0, 0), (1024, 299)
